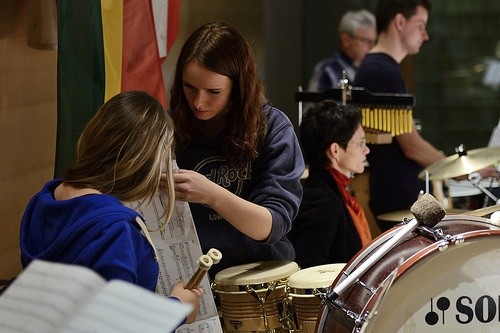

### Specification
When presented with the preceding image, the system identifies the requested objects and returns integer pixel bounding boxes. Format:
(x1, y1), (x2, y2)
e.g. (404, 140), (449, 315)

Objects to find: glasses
(349, 34), (376, 46)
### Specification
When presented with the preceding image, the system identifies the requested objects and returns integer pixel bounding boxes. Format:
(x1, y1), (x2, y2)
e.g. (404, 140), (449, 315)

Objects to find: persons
(301, 9), (377, 113)
(158, 20), (306, 284)
(285, 101), (371, 272)
(19, 90), (205, 332)
(352, 0), (449, 233)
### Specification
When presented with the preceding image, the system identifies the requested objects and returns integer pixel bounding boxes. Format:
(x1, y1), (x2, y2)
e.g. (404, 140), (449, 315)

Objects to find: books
(122, 159), (222, 332)
(0, 259), (196, 333)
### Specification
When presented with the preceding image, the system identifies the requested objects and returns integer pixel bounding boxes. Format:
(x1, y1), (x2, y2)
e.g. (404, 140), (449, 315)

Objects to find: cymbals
(417, 145), (500, 181)
(453, 204), (500, 221)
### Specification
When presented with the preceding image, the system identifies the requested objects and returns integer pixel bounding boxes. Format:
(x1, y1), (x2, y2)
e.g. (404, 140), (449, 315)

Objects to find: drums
(310, 216), (499, 333)
(285, 262), (349, 333)
(209, 258), (300, 333)
